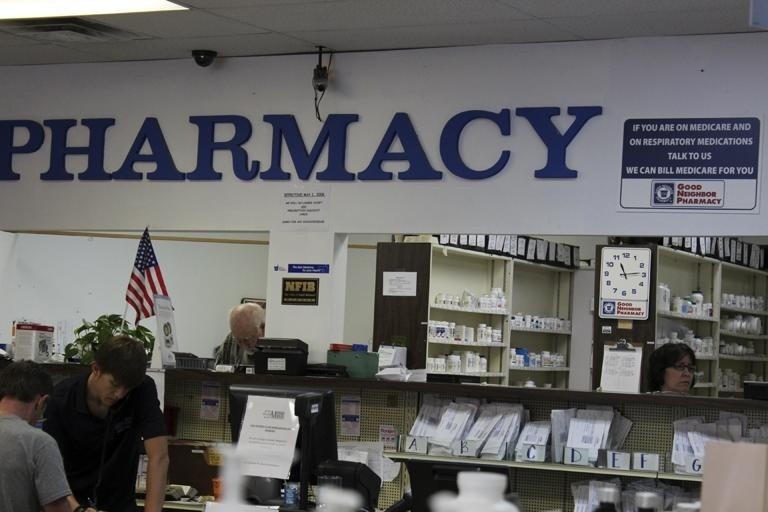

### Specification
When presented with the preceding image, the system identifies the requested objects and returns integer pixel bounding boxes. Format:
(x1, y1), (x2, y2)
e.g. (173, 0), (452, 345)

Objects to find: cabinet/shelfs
(593, 245), (767, 400)
(372, 241), (574, 395)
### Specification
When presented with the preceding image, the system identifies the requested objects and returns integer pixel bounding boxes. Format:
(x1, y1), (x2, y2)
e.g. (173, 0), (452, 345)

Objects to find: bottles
(427, 351), (487, 373)
(670, 328), (702, 353)
(446, 471), (520, 512)
(721, 291), (766, 392)
(634, 491), (660, 512)
(428, 320), (501, 342)
(509, 347), (564, 368)
(434, 287), (505, 309)
(658, 283), (713, 318)
(591, 487), (619, 512)
(511, 312), (571, 332)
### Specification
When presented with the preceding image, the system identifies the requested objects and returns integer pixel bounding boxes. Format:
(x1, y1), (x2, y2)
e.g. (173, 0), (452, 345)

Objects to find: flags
(125, 229), (175, 326)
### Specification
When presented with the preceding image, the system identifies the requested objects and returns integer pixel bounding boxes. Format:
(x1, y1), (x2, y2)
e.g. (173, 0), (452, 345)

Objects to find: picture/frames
(241, 297), (267, 309)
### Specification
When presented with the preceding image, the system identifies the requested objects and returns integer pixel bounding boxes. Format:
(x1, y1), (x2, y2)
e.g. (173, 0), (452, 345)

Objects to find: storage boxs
(12, 322), (54, 365)
(327, 349), (378, 381)
(254, 336), (309, 376)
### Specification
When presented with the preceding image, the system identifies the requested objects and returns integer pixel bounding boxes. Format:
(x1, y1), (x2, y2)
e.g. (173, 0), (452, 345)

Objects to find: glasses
(37, 392), (50, 424)
(673, 364), (696, 374)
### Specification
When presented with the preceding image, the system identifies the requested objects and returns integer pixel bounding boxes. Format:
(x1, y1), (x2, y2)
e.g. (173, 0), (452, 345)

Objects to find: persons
(42, 336), (169, 512)
(1, 359), (83, 512)
(215, 303), (265, 369)
(647, 343), (696, 394)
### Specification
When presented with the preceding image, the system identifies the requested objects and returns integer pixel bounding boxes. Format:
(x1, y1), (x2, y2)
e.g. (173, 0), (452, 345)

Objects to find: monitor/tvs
(229, 386), (338, 481)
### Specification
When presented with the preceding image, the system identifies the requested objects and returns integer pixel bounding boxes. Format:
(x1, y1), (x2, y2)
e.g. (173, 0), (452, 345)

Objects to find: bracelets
(73, 505), (86, 511)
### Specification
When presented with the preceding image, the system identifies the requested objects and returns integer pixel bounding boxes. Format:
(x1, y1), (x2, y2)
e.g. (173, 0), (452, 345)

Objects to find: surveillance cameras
(312, 64), (328, 91)
(192, 50), (217, 67)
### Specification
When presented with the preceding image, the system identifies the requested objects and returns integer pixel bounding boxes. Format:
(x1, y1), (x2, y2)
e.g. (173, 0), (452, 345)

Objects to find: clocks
(598, 246), (652, 322)
